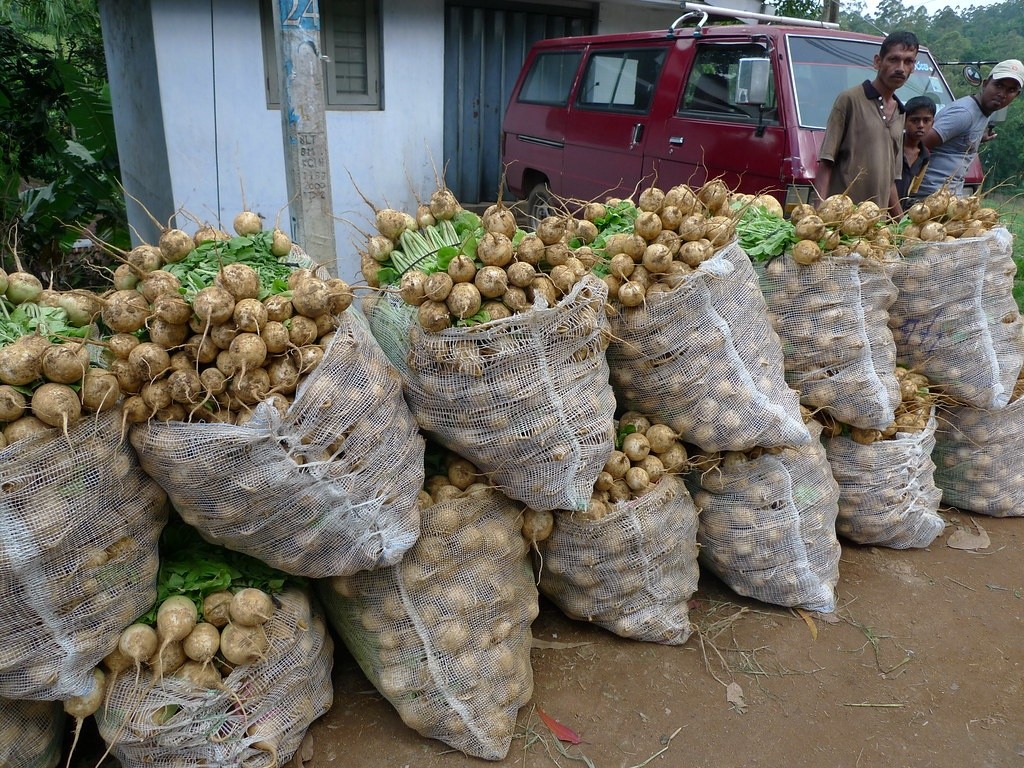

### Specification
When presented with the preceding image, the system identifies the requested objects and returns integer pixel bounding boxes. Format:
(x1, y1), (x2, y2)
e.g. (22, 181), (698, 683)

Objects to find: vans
(500, 2), (1009, 233)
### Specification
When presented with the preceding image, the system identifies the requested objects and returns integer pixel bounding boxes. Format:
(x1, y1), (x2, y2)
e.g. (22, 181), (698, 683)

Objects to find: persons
(887, 96), (938, 222)
(917, 58), (1024, 203)
(812, 31), (919, 223)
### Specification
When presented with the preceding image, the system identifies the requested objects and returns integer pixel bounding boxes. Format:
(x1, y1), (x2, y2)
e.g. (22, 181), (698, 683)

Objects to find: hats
(987, 59), (1024, 88)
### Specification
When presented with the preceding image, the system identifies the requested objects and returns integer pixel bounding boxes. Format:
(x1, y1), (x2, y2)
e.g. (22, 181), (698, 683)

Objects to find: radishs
(0, 169), (1024, 768)
